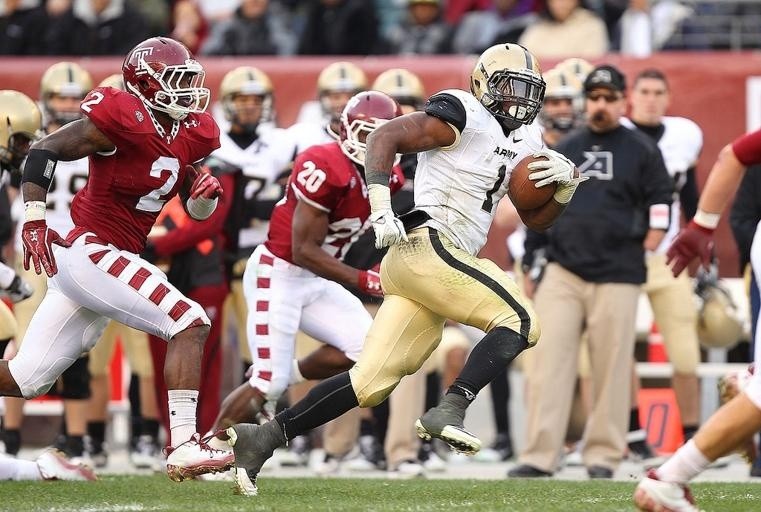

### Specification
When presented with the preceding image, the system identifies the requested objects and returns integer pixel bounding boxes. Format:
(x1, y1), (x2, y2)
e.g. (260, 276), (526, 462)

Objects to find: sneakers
(508, 464), (551, 479)
(588, 466), (613, 479)
(129, 434), (160, 469)
(37, 448), (96, 481)
(414, 407), (482, 456)
(227, 423), (272, 499)
(633, 469), (698, 512)
(163, 433), (237, 482)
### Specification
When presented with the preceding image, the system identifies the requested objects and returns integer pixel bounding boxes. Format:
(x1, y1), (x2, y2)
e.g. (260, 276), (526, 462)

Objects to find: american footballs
(509, 154), (556, 210)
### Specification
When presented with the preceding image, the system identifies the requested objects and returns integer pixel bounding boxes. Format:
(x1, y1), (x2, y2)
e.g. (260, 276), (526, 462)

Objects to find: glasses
(588, 95), (617, 103)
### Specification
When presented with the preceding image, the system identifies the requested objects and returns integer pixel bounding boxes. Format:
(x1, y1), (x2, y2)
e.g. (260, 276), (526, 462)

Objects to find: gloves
(186, 165), (225, 200)
(667, 208), (720, 279)
(367, 183), (410, 251)
(360, 260), (386, 300)
(526, 149), (590, 205)
(21, 201), (72, 278)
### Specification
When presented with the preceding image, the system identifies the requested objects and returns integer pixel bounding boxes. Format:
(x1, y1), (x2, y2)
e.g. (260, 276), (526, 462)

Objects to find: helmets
(219, 66), (275, 124)
(337, 92), (402, 169)
(372, 68), (429, 104)
(122, 37), (211, 121)
(0, 90), (42, 181)
(554, 57), (597, 83)
(697, 280), (752, 353)
(42, 61), (92, 126)
(470, 43), (547, 126)
(317, 61), (368, 122)
(542, 69), (583, 101)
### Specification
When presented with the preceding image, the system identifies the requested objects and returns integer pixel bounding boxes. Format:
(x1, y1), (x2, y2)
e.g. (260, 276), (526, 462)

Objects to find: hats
(584, 66), (625, 94)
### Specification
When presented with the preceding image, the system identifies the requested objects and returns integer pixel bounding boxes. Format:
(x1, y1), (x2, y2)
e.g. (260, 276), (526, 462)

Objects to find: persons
(1, 2), (761, 497)
(632, 128), (761, 511)
